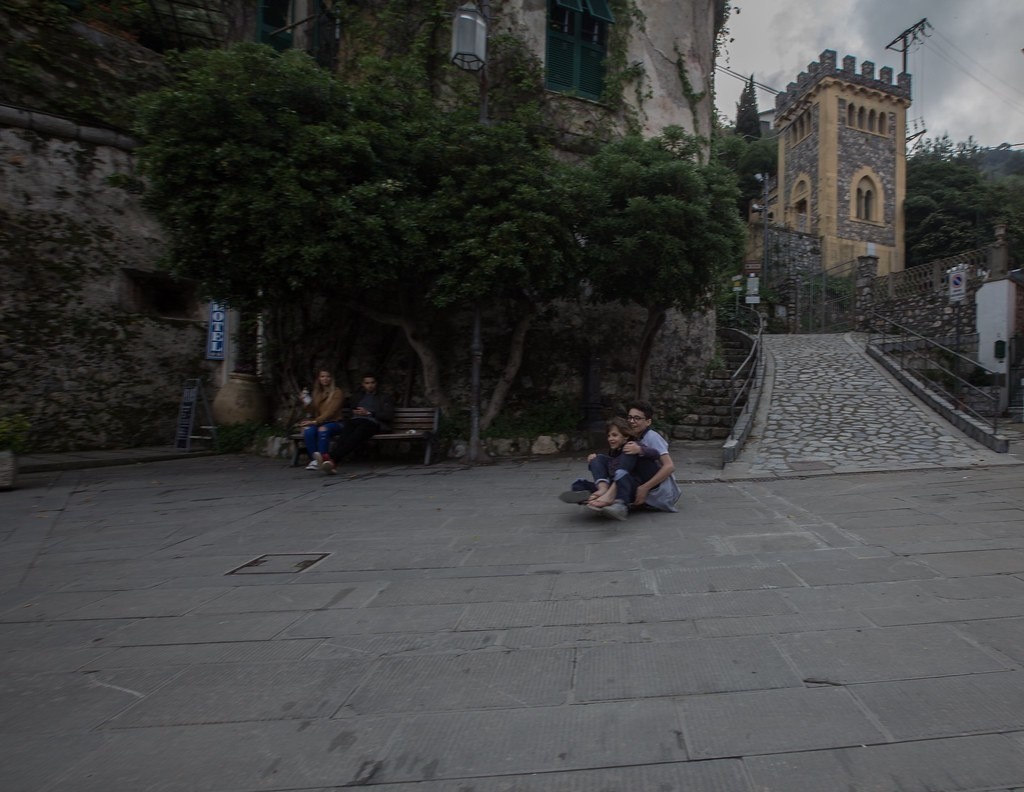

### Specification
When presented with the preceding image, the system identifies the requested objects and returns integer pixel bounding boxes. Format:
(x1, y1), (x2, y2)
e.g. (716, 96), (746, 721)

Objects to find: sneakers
(313, 452), (324, 463)
(305, 460), (320, 471)
(601, 504), (629, 521)
(558, 490), (591, 503)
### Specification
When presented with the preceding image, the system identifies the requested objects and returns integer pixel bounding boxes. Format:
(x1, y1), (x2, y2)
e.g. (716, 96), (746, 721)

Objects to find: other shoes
(327, 466), (337, 475)
(321, 459), (334, 473)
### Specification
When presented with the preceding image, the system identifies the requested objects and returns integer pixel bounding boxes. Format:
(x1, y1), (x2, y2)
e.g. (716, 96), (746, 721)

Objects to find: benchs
(291, 407), (440, 466)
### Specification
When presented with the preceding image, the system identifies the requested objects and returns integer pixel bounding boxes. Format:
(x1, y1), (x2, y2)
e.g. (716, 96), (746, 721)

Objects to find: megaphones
(751, 204), (763, 213)
(754, 173), (764, 183)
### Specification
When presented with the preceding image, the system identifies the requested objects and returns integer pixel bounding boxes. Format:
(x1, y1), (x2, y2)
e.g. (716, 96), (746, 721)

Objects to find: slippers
(582, 493), (605, 507)
(586, 498), (609, 511)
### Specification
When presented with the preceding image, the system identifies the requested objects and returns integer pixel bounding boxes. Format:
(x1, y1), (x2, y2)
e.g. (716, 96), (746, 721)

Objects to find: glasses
(363, 381), (376, 384)
(626, 414), (646, 421)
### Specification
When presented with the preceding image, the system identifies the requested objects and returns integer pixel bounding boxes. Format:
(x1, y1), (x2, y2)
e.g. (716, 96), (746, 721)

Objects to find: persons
(320, 372), (395, 476)
(561, 401), (683, 522)
(300, 363), (343, 470)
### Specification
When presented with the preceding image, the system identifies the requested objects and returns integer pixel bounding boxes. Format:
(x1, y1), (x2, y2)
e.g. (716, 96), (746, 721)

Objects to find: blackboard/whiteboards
(175, 379), (218, 439)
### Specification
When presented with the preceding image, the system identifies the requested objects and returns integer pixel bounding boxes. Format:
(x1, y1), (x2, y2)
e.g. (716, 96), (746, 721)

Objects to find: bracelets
(367, 411), (371, 415)
